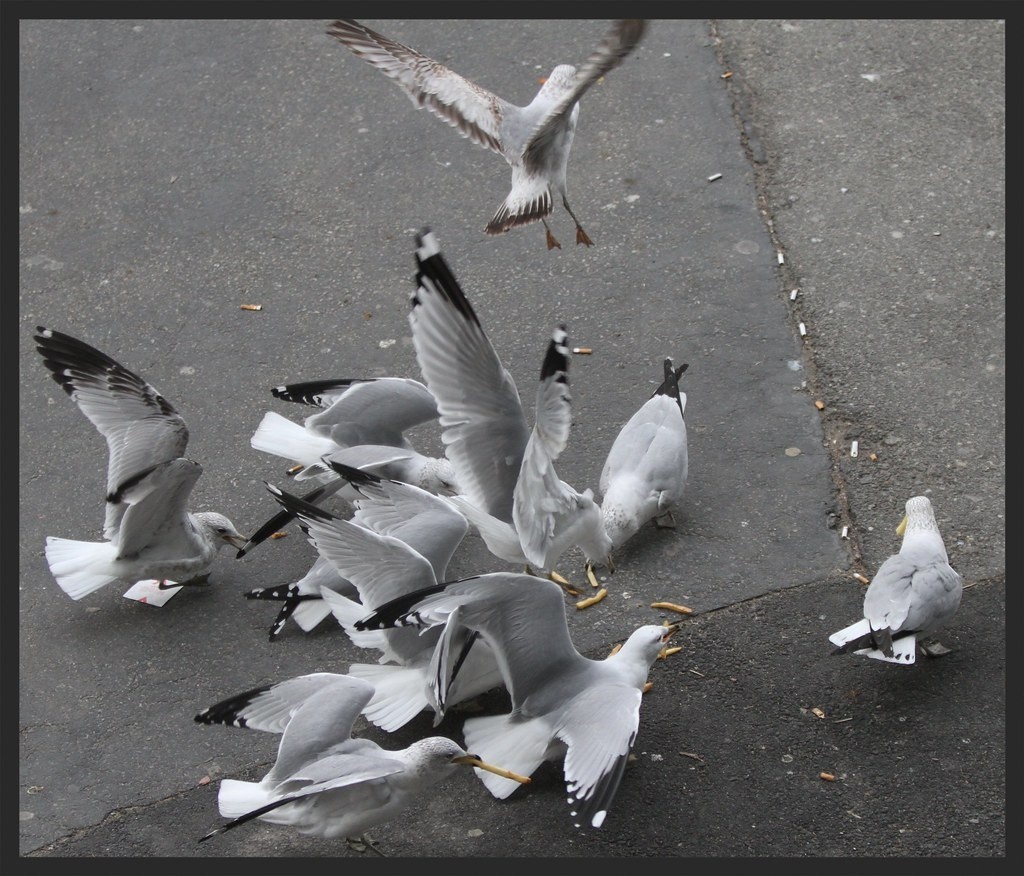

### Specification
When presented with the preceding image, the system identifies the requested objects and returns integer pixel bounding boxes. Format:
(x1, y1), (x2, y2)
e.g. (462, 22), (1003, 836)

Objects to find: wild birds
(33, 228), (692, 863)
(324, 19), (649, 251)
(828, 496), (963, 664)
